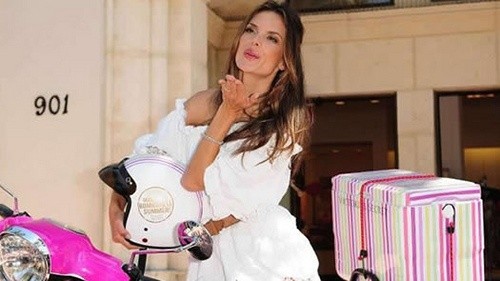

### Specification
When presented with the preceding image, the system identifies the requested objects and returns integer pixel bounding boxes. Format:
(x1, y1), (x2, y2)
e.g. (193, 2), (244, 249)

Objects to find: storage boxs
(330, 169), (485, 281)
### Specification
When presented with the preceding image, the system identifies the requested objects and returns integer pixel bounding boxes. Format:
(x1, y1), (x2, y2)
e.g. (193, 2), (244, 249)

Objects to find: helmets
(99, 154), (203, 248)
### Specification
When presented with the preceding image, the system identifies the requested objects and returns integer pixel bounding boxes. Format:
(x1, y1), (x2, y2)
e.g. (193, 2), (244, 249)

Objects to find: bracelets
(200, 132), (224, 145)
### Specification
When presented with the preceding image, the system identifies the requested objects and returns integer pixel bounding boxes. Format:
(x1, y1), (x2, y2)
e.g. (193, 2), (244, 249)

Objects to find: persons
(108, 0), (320, 280)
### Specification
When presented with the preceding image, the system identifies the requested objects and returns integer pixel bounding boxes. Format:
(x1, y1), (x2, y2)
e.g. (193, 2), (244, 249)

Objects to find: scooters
(0, 161), (214, 279)
